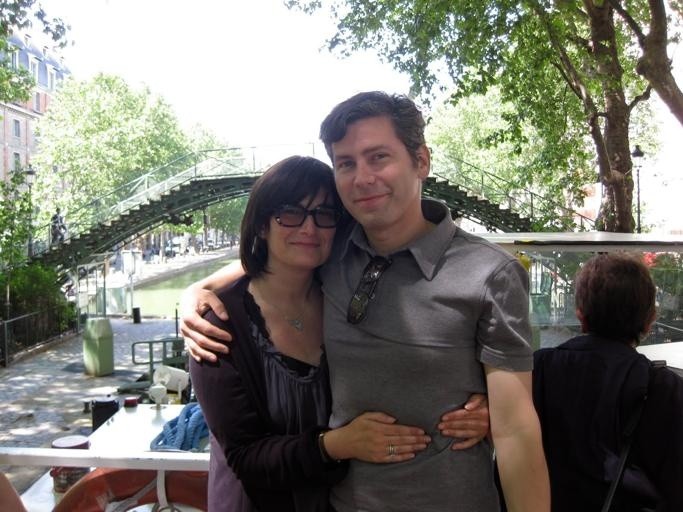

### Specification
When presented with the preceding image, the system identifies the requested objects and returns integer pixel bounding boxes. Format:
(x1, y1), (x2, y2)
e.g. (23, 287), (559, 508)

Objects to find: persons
(177, 90), (556, 511)
(48, 207), (67, 250)
(187, 154), (493, 511)
(530, 250), (683, 511)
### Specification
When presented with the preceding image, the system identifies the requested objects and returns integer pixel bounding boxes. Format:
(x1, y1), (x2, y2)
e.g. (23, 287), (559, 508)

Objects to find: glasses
(348, 255), (390, 323)
(269, 202), (345, 229)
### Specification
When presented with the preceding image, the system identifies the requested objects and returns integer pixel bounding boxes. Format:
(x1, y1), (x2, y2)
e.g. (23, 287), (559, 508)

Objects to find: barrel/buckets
(52, 435), (90, 500)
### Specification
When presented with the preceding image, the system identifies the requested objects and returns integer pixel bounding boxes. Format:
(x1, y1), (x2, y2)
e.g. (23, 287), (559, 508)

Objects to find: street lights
(24, 163), (37, 259)
(632, 143), (646, 232)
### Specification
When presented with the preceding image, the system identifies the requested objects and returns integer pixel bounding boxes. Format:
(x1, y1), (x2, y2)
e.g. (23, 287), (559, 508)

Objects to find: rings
(388, 442), (397, 458)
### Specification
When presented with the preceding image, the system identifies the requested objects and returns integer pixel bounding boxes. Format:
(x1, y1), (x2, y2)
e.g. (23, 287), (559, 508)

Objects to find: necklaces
(256, 280), (313, 331)
